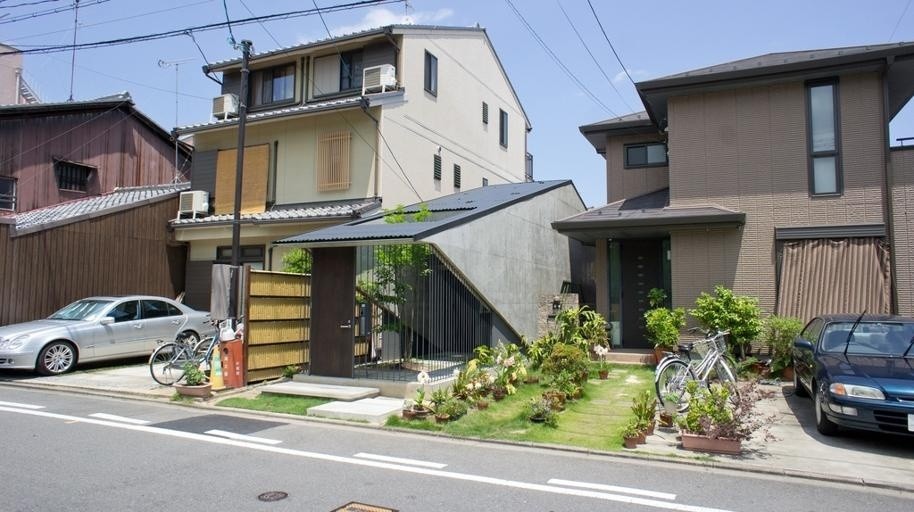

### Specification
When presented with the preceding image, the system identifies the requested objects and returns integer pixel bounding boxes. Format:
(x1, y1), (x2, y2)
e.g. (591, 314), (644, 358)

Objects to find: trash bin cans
(610, 321), (622, 349)
(221, 340), (244, 387)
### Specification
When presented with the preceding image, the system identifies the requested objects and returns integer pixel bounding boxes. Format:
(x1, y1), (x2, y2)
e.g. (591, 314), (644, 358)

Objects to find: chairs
(825, 330), (855, 353)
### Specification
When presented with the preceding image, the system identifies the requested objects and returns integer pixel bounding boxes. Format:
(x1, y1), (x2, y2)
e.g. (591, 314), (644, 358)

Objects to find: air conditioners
(362, 63), (397, 92)
(213, 93), (238, 121)
(178, 190), (209, 214)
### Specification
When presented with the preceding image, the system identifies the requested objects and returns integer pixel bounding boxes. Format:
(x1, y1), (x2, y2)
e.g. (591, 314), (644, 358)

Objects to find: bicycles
(150, 314), (245, 386)
(654, 325), (741, 415)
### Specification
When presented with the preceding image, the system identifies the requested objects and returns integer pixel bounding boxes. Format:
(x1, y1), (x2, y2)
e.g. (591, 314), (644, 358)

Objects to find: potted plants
(396, 303), (609, 426)
(623, 285), (795, 455)
(173, 360), (212, 398)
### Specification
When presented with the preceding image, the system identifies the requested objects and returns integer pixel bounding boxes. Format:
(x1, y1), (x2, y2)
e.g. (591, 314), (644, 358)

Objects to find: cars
(792, 314), (914, 439)
(1, 296), (219, 374)
